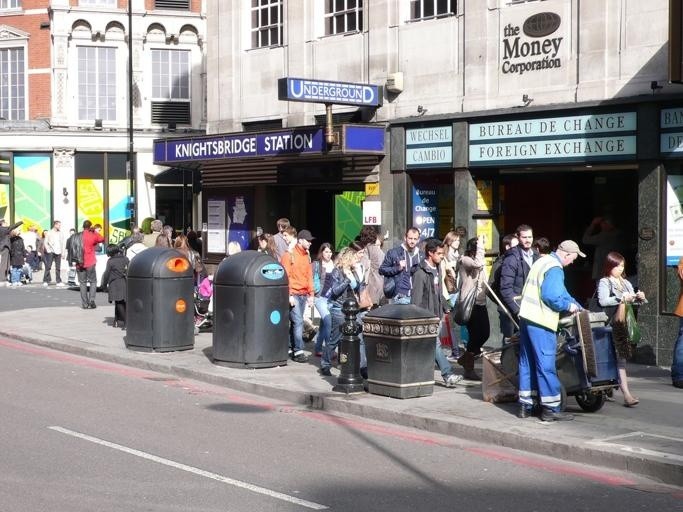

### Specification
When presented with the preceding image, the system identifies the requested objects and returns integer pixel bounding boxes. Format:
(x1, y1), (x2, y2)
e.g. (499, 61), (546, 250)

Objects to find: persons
(672, 256), (683, 387)
(487, 234), (520, 305)
(249, 219), (490, 386)
(533, 238), (550, 256)
(0, 220), (214, 335)
(584, 216), (620, 296)
(597, 252), (648, 406)
(501, 224), (541, 361)
(518, 240), (590, 421)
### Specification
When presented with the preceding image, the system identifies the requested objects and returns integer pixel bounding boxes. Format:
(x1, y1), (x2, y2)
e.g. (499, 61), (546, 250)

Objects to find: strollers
(193, 290), (213, 337)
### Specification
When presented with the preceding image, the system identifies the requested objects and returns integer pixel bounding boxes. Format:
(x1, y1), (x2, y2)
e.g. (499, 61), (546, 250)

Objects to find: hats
(298, 230), (316, 242)
(557, 240), (587, 258)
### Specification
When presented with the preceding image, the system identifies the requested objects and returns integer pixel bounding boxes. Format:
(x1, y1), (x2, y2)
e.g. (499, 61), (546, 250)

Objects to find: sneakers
(443, 353), (638, 422)
(288, 347), (368, 379)
(0, 282), (127, 330)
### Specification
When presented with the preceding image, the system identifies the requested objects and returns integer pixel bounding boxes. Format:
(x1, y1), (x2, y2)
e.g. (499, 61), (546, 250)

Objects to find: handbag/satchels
(588, 294), (618, 325)
(314, 273), (321, 294)
(383, 276), (396, 298)
(453, 288), (476, 326)
(444, 274), (458, 294)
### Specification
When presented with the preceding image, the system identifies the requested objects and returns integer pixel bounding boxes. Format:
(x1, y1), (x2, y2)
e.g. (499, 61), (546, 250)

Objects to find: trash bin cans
(211, 249), (289, 367)
(124, 248), (195, 352)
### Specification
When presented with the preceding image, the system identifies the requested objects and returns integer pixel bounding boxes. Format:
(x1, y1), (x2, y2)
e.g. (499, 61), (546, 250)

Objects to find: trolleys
(480, 312), (620, 415)
